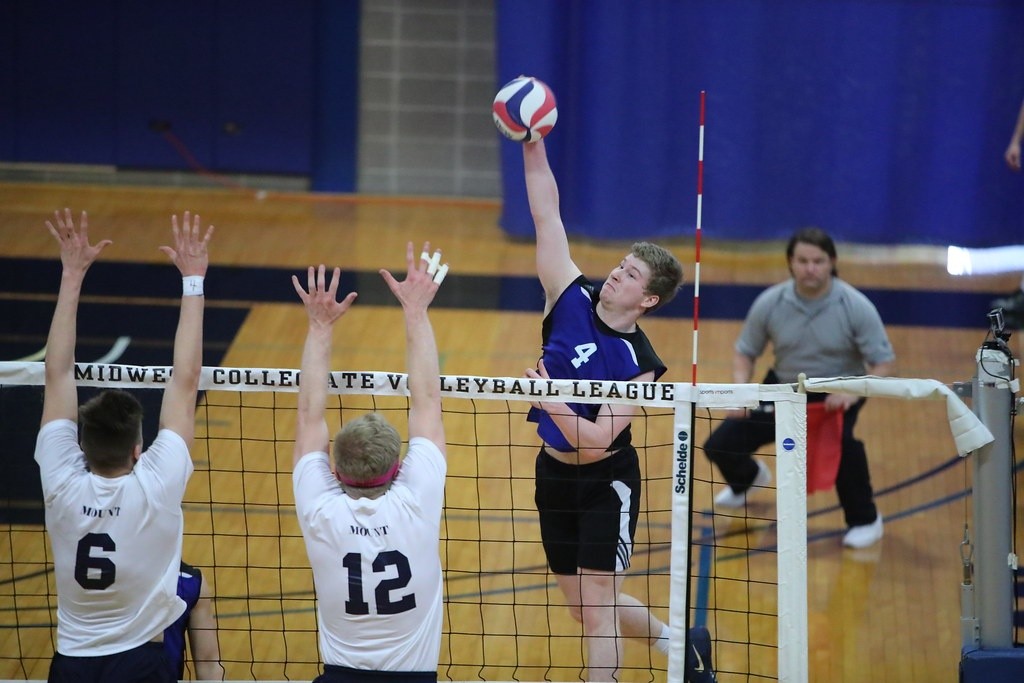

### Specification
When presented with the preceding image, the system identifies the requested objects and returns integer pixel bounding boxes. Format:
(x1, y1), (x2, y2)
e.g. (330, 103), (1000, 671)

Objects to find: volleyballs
(490, 74), (559, 145)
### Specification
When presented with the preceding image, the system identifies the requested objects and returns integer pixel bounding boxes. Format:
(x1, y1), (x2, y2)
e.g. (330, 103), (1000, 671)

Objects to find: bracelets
(179, 276), (204, 296)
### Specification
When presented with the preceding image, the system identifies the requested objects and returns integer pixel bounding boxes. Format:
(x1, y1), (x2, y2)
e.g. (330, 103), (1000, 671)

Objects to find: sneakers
(685, 626), (716, 683)
(714, 459), (772, 508)
(842, 512), (884, 549)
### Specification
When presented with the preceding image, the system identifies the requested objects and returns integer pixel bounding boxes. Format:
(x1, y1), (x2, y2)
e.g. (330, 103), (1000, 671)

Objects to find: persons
(704, 228), (895, 548)
(517, 74), (717, 683)
(33, 207), (224, 683)
(292, 241), (449, 683)
